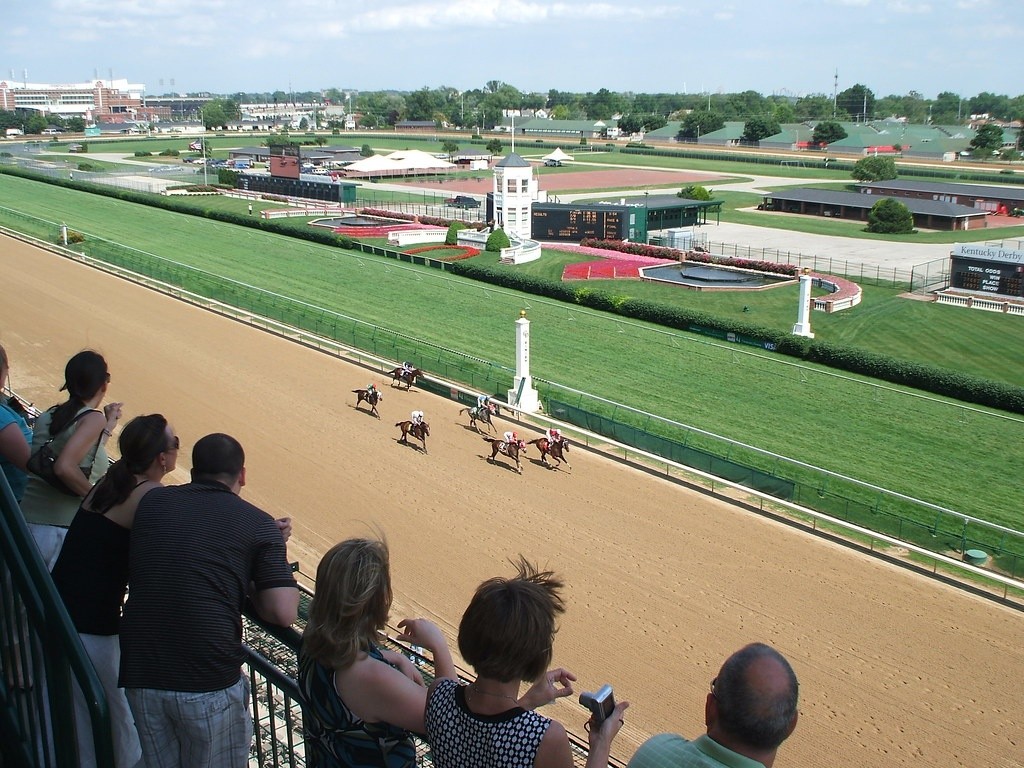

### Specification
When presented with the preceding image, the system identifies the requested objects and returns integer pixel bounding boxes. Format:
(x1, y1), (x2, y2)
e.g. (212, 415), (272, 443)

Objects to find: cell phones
(290, 561), (299, 572)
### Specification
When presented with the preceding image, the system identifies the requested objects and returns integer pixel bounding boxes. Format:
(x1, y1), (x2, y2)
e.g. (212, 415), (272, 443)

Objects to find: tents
(541, 147), (574, 168)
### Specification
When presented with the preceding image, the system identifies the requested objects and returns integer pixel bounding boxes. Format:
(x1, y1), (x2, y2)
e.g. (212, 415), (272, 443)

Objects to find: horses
(459, 403), (497, 435)
(351, 388), (383, 420)
(387, 368), (423, 392)
(482, 436), (527, 475)
(525, 437), (572, 471)
(395, 421), (431, 455)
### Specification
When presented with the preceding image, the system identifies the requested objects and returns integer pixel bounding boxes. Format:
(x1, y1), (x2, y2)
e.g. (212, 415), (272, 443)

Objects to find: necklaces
(472, 682), (518, 705)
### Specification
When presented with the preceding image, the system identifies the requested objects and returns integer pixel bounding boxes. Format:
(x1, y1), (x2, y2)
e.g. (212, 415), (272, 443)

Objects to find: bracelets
(103, 429), (112, 437)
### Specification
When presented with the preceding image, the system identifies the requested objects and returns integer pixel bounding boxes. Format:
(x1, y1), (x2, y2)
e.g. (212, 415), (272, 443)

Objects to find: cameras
(578, 683), (617, 729)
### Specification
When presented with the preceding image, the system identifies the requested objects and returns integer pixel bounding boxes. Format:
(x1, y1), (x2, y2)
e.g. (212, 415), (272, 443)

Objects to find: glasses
(710, 677), (719, 700)
(105, 373), (110, 382)
(159, 436), (179, 454)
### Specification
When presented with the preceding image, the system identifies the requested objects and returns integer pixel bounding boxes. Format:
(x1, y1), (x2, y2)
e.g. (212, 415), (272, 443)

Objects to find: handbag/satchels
(26, 409), (105, 497)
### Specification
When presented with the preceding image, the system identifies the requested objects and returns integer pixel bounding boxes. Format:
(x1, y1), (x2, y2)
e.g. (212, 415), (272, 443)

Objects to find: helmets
(373, 384), (376, 387)
(557, 429), (561, 434)
(419, 411), (423, 416)
(486, 396), (490, 400)
(513, 432), (517, 437)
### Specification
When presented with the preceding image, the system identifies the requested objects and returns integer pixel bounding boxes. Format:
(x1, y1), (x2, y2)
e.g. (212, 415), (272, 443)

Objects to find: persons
(117, 433), (299, 768)
(504, 432), (517, 447)
(400, 360), (413, 376)
(546, 427), (561, 450)
(70, 171), (73, 180)
(297, 538), (459, 768)
(423, 554), (630, 768)
(366, 383), (377, 398)
(625, 642), (798, 768)
(830, 208), (840, 217)
(412, 411), (423, 430)
(789, 206), (793, 213)
(248, 203), (252, 216)
(410, 643), (425, 666)
(477, 395), (490, 416)
(0, 344), (123, 695)
(41, 414), (179, 768)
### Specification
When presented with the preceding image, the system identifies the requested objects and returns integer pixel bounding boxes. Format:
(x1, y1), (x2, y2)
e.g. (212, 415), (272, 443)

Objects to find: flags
(190, 138), (202, 150)
